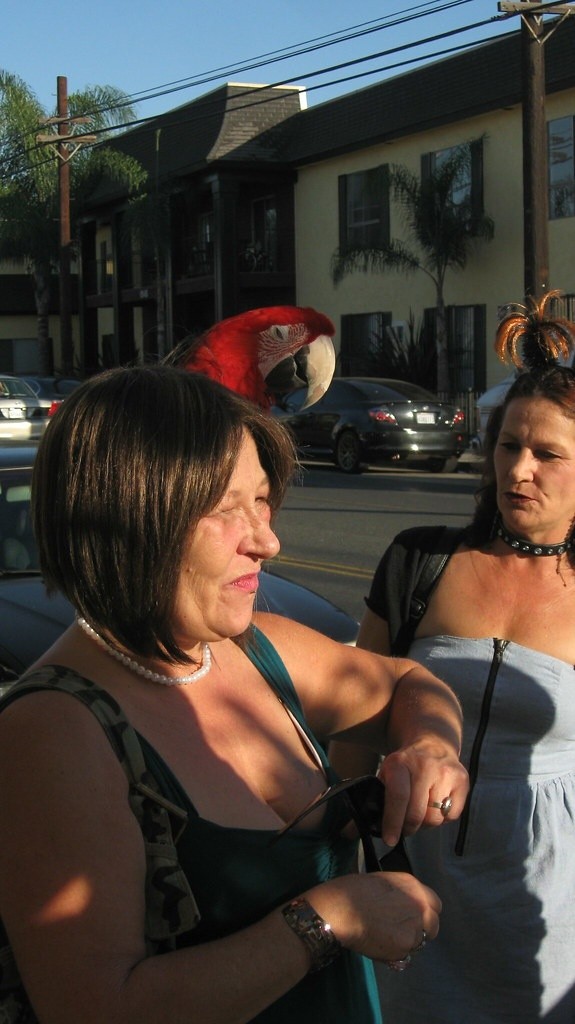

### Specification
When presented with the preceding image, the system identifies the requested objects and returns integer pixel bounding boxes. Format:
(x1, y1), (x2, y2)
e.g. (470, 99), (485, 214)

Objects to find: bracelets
(282, 899), (340, 972)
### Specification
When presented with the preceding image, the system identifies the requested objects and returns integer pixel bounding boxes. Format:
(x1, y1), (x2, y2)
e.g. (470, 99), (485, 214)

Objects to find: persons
(0, 366), (471, 1024)
(326, 291), (575, 1024)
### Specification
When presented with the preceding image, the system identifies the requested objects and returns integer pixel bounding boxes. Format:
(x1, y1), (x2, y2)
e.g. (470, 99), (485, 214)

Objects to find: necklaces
(75, 611), (212, 686)
(493, 511), (572, 556)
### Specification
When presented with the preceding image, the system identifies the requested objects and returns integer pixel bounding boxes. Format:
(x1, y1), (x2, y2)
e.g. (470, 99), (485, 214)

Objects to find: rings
(388, 956), (410, 971)
(429, 797), (453, 816)
(412, 932), (427, 952)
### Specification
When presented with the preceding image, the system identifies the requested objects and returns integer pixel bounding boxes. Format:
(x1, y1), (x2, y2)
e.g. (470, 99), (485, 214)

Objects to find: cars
(0, 375), (62, 441)
(1, 443), (363, 701)
(23, 377), (83, 417)
(273, 377), (466, 474)
